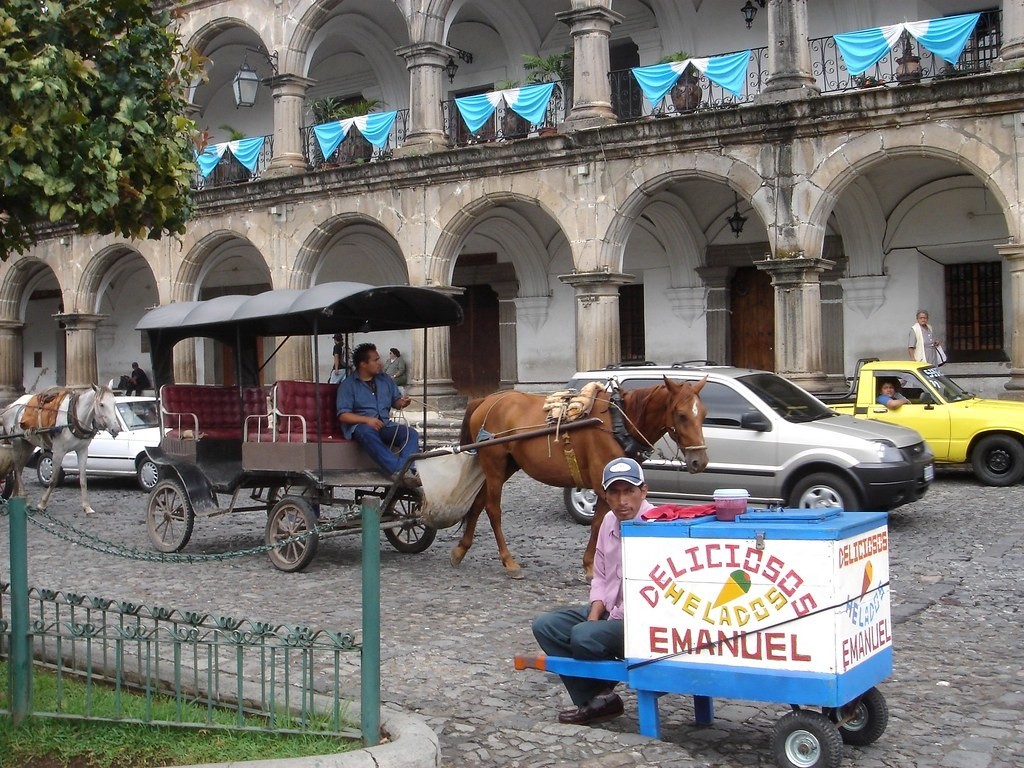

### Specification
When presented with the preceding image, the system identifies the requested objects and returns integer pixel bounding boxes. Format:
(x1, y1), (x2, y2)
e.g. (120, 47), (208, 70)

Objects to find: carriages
(135, 281), (708, 581)
(0, 377), (121, 518)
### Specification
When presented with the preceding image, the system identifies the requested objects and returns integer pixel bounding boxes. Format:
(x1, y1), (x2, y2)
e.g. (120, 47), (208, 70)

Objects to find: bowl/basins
(713, 489), (750, 520)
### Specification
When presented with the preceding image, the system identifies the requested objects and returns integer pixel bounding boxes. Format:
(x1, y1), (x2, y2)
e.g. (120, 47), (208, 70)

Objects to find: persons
(382, 348), (408, 386)
(330, 334), (347, 384)
(336, 343), (423, 488)
(908, 309), (943, 369)
(531, 458), (659, 725)
(876, 377), (912, 409)
(125, 362), (150, 396)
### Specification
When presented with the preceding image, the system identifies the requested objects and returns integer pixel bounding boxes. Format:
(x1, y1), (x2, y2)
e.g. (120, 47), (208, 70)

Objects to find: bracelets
(394, 375), (397, 378)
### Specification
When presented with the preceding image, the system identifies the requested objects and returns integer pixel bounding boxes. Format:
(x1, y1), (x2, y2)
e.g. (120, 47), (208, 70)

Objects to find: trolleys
(515, 507), (893, 768)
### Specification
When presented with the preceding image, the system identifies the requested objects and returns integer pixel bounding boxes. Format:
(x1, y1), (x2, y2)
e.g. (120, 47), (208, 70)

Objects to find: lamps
(233, 43), (280, 107)
(740, 0), (766, 30)
(445, 42), (473, 83)
(725, 189), (748, 240)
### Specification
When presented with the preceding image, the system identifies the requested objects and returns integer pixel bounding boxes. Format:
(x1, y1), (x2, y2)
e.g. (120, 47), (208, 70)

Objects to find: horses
(445, 374), (709, 578)
(0, 379), (119, 518)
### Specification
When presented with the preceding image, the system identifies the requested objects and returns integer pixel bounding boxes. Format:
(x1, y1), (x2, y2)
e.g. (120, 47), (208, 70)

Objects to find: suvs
(563, 360), (935, 525)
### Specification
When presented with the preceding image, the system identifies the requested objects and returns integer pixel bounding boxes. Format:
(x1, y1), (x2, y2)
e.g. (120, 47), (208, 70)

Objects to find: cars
(26, 395), (173, 493)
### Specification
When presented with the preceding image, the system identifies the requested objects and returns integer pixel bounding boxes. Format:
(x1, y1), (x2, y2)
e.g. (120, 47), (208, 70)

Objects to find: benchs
(113, 386), (156, 396)
(160, 380), (354, 458)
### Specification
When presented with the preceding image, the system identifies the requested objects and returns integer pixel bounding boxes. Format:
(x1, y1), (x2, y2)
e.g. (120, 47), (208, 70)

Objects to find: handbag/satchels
(935, 345), (946, 367)
(329, 369), (346, 384)
(118, 375), (131, 390)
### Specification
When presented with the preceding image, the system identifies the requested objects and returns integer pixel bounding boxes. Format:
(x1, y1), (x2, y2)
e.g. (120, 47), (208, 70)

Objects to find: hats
(332, 334), (342, 339)
(601, 457), (645, 491)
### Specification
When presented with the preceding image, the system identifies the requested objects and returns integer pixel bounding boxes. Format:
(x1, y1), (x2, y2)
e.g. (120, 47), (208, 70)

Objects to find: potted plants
(335, 99), (377, 163)
(894, 19), (922, 83)
(308, 95), (340, 172)
(518, 50), (573, 137)
(218, 124), (249, 185)
(654, 52), (701, 114)
(856, 75), (886, 88)
(492, 78), (532, 138)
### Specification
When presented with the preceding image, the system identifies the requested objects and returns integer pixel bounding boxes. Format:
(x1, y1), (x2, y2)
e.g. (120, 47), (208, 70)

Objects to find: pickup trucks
(747, 358), (1024, 488)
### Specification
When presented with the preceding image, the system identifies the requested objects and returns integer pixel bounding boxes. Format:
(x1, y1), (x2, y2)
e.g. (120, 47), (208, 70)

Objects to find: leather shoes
(559, 693), (624, 725)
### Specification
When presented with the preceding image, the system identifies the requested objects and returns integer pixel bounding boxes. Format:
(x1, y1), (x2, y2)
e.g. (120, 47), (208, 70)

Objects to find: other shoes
(391, 468), (422, 487)
